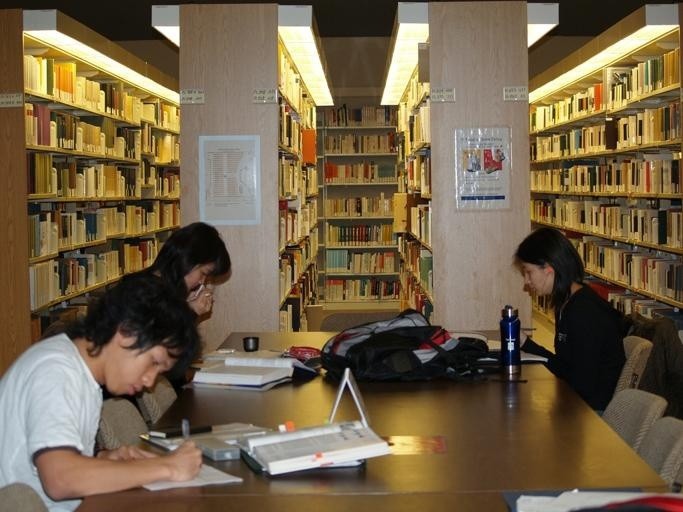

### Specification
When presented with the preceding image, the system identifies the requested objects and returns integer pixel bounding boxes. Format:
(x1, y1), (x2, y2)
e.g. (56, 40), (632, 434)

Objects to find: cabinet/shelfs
(529, 3), (683, 340)
(180, 15), (318, 357)
(392, 0), (530, 331)
(318, 103), (396, 302)
(0, 31), (180, 379)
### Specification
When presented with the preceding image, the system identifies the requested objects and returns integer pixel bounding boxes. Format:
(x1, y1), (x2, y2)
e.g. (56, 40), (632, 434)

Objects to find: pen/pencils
(182, 419), (190, 442)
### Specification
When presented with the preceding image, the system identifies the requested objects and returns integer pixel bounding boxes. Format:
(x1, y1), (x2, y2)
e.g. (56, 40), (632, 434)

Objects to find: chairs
(0, 483), (50, 512)
(599, 388), (668, 452)
(96, 399), (148, 452)
(615, 334), (654, 393)
(636, 416), (682, 487)
(137, 377), (177, 426)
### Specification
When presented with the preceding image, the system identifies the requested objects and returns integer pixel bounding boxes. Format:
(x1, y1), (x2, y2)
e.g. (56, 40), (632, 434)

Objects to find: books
(140, 420), (391, 476)
(193, 350), (316, 391)
(279, 52), (434, 332)
(529, 48), (683, 323)
(24, 54), (181, 310)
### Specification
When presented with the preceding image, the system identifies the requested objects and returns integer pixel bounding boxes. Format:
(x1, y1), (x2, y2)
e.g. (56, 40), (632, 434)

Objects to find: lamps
(23, 8), (180, 104)
(378, 1), (429, 106)
(150, 5), (179, 49)
(529, 3), (679, 104)
(278, 4), (336, 107)
(526, 3), (560, 48)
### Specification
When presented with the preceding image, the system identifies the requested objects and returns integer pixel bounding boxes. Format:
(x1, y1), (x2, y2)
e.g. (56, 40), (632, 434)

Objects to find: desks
(72, 331), (669, 512)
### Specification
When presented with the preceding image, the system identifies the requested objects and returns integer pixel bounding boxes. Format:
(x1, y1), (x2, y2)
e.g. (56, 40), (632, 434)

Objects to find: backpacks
(318, 307), (490, 387)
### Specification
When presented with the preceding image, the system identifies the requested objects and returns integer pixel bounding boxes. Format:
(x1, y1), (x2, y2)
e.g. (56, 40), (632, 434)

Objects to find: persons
(140, 222), (230, 390)
(515, 228), (631, 416)
(0, 273), (203, 512)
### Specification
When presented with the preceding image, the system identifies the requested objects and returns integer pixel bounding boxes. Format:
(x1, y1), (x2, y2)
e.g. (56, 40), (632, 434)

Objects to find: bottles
(498, 305), (523, 375)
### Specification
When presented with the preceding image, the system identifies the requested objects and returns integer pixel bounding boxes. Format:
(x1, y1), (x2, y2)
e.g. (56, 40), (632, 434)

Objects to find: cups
(242, 336), (261, 352)
